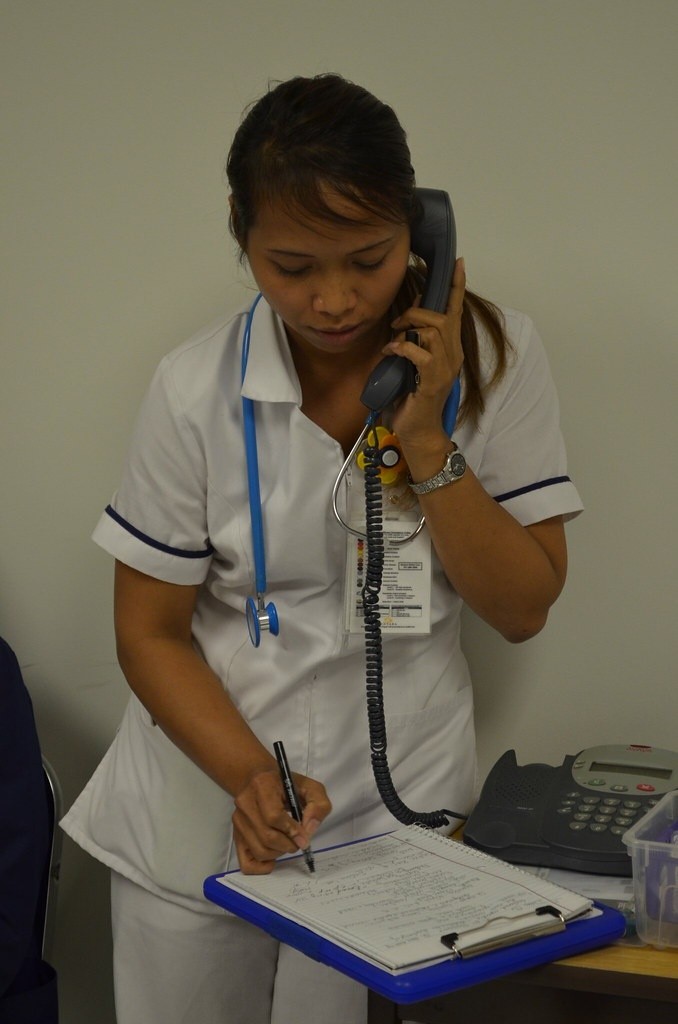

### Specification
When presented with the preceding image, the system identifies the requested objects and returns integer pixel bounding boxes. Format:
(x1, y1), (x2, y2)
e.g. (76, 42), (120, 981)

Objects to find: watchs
(407, 447), (468, 495)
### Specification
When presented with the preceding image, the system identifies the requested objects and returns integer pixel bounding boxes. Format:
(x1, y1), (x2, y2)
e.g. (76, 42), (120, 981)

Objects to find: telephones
(359, 186), (457, 413)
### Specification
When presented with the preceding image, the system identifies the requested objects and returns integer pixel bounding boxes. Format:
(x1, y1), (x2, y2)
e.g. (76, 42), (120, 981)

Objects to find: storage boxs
(621, 790), (678, 951)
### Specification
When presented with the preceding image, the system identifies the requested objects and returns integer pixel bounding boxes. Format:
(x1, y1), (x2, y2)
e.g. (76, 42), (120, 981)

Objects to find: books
(216, 822), (594, 975)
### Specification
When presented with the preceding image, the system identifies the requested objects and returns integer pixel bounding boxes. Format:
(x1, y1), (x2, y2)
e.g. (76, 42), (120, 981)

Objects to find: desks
(369, 822), (678, 1003)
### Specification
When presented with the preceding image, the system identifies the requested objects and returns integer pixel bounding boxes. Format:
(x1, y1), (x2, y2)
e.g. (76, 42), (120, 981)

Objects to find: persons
(57, 75), (585, 1024)
